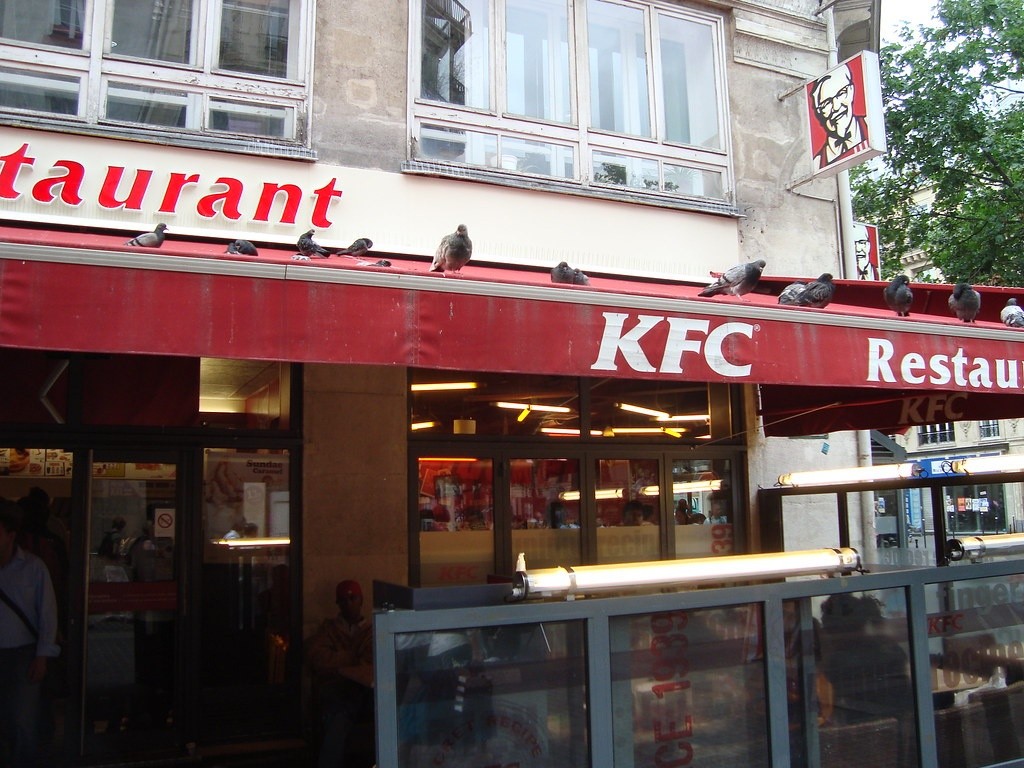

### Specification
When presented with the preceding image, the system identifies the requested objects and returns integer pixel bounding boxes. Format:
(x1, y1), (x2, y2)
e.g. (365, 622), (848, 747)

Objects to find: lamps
(453, 419), (476, 435)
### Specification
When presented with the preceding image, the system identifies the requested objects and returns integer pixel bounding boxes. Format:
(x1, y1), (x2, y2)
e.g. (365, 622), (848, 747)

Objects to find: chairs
(818, 716), (898, 768)
(672, 739), (768, 768)
(1001, 681), (1024, 758)
(913, 702), (995, 768)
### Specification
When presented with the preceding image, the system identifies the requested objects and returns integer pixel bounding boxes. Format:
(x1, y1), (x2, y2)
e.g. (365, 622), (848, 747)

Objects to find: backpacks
(99, 530), (118, 555)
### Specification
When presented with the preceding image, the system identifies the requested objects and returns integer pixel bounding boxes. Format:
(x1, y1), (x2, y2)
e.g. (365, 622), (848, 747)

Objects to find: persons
(98, 517), (154, 564)
(820, 593), (913, 725)
(222, 515), (258, 541)
(307, 580), (373, 768)
(0, 496), (70, 768)
(418, 492), (729, 531)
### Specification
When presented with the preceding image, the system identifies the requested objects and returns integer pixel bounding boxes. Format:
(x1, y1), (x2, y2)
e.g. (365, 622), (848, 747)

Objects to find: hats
(335, 581), (364, 602)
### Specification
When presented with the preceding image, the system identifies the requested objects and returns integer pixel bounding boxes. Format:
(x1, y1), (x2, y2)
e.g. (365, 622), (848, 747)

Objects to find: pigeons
(123, 222), (168, 248)
(1000, 298), (1024, 328)
(697, 259), (766, 303)
(337, 237), (373, 259)
(550, 261), (590, 286)
(296, 229), (331, 258)
(777, 273), (834, 309)
(947, 283), (981, 323)
(883, 275), (914, 318)
(429, 223), (473, 279)
(226, 240), (258, 255)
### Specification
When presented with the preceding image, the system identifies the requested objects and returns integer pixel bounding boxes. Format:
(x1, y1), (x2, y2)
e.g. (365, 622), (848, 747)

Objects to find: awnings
(1, 226), (1024, 438)
